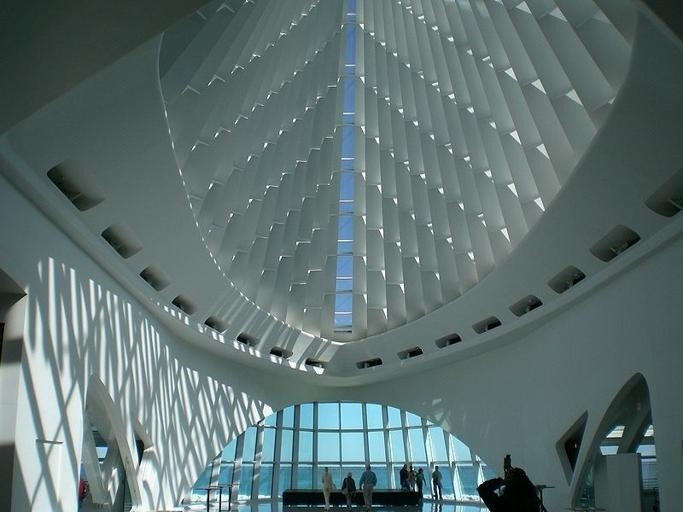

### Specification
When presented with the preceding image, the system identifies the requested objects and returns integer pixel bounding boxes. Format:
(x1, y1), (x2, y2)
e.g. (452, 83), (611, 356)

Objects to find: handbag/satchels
(342, 488), (348, 495)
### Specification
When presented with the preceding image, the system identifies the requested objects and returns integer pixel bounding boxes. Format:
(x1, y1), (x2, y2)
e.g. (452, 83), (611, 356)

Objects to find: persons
(431, 465), (443, 500)
(407, 465), (417, 490)
(414, 468), (426, 492)
(399, 464), (411, 489)
(476, 467), (542, 512)
(358, 463), (376, 512)
(340, 471), (356, 512)
(320, 467), (336, 512)
